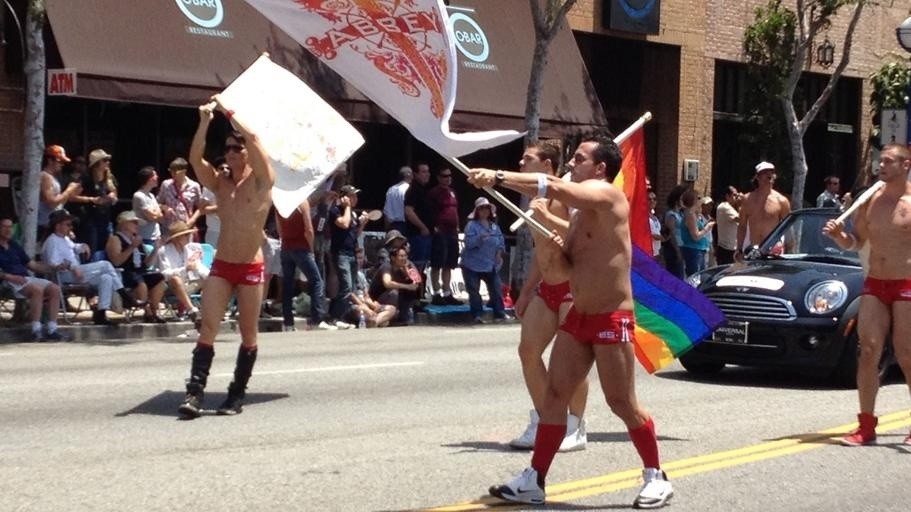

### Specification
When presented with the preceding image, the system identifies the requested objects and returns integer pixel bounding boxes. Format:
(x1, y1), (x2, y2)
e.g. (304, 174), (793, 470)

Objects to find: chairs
(32, 325), (73, 342)
(179, 381), (204, 417)
(142, 307), (199, 322)
(509, 408), (540, 450)
(284, 319), (355, 331)
(556, 411), (587, 451)
(632, 467), (675, 509)
(841, 412), (878, 445)
(488, 466), (543, 505)
(122, 300), (148, 308)
(94, 318), (119, 326)
(216, 384), (244, 414)
(473, 314), (516, 324)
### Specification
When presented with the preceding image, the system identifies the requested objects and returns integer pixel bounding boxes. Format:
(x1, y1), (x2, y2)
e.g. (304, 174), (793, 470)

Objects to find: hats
(665, 203), (901, 385)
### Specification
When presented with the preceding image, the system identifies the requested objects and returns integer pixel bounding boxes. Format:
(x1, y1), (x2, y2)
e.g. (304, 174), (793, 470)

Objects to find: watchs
(493, 170), (505, 189)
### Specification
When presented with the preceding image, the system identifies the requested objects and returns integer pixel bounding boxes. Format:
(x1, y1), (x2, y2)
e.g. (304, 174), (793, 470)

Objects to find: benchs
(610, 126), (728, 374)
(220, 55), (363, 216)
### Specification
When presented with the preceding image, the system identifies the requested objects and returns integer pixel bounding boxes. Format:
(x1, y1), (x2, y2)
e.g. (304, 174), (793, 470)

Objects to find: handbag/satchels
(225, 109), (234, 122)
(843, 234), (856, 252)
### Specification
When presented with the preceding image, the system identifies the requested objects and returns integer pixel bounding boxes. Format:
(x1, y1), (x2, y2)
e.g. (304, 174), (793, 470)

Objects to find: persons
(37, 146), (82, 262)
(132, 168), (163, 247)
(430, 165), (465, 306)
(403, 164), (436, 278)
(180, 94), (273, 420)
(647, 161), (852, 279)
(107, 211), (169, 324)
(509, 140), (588, 453)
(469, 136), (672, 511)
(823, 142), (911, 449)
(2, 218), (70, 342)
(156, 160), (203, 243)
(68, 149), (118, 261)
(157, 220), (214, 322)
(46, 210), (148, 325)
(460, 198), (508, 325)
(264, 185), (422, 332)
(199, 188), (222, 247)
(384, 167), (412, 236)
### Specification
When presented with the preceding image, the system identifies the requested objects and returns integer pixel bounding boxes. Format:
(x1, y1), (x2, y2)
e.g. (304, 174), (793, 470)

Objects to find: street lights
(486, 283), (513, 308)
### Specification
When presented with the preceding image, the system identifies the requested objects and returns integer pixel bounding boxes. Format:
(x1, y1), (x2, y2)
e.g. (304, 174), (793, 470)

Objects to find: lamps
(94, 250), (147, 322)
(170, 243), (217, 318)
(144, 243), (189, 321)
(35, 251), (95, 325)
(1, 269), (49, 326)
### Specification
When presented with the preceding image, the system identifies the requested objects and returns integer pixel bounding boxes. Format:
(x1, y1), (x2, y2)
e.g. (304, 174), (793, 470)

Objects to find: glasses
(810, 32), (836, 70)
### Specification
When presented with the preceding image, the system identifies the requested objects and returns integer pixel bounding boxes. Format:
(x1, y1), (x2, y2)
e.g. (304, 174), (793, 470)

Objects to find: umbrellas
(432, 291), (465, 305)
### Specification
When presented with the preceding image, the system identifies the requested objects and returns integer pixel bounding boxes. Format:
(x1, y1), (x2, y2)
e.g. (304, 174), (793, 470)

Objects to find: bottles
(47, 209), (77, 225)
(166, 220), (199, 242)
(338, 184), (361, 198)
(754, 160), (775, 173)
(46, 144), (70, 163)
(117, 209), (147, 227)
(88, 148), (113, 167)
(699, 196), (714, 206)
(384, 230), (407, 246)
(467, 197), (497, 219)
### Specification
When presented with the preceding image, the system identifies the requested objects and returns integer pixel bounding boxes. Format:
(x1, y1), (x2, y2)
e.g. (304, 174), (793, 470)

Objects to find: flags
(220, 55), (363, 216)
(610, 126), (728, 374)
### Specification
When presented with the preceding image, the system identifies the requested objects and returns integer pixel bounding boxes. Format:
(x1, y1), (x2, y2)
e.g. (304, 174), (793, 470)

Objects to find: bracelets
(843, 234), (856, 252)
(225, 109), (234, 122)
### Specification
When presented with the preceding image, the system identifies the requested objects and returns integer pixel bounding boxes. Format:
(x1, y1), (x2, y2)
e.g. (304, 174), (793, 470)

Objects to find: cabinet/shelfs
(358, 310), (366, 330)
(408, 308), (414, 323)
